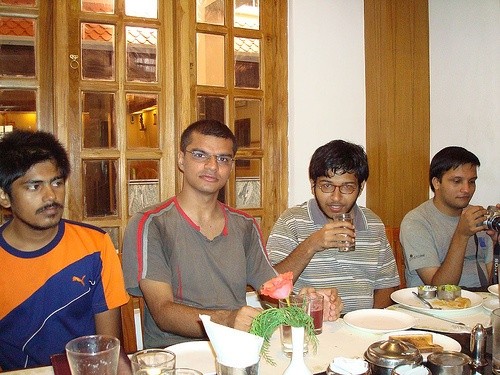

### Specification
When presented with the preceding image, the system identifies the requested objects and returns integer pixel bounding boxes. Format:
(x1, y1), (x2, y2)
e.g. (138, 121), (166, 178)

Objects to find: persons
(0, 129), (133, 375)
(119, 118), (344, 349)
(401, 146), (500, 290)
(264, 140), (400, 322)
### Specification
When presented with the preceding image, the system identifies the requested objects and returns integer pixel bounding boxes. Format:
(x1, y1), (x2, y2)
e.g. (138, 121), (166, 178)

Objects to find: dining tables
(115, 288), (500, 375)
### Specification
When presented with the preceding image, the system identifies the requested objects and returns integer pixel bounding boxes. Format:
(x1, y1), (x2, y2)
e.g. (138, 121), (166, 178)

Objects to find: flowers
(248, 272), (319, 366)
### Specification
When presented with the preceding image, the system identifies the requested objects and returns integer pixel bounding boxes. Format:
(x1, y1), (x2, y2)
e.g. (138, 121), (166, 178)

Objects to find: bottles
(469, 323), (488, 367)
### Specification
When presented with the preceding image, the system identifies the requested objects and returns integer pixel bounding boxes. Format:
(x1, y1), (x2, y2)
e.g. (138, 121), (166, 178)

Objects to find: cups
(215, 355), (261, 375)
(299, 292), (324, 335)
(490, 308), (500, 375)
(66, 335), (120, 375)
(325, 339), (472, 375)
(279, 296), (309, 357)
(159, 367), (203, 375)
(131, 348), (176, 375)
(333, 212), (355, 252)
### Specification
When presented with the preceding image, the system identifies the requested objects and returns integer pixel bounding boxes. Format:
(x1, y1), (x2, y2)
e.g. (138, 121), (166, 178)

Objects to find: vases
(281, 326), (315, 375)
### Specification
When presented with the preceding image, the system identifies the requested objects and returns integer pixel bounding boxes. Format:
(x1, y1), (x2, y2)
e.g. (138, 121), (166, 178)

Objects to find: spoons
(412, 291), (442, 310)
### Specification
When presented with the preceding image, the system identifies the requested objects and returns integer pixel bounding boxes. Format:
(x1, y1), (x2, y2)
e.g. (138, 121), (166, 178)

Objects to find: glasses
(315, 180), (361, 194)
(182, 149), (235, 167)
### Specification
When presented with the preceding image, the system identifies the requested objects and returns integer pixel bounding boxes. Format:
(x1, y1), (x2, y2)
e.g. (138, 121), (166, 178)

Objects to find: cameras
(476, 206), (500, 231)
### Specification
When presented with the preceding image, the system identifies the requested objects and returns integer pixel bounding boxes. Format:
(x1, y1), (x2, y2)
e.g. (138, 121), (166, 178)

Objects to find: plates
(343, 309), (416, 333)
(482, 284), (499, 312)
(163, 340), (217, 375)
(390, 286), (484, 312)
(373, 329), (461, 362)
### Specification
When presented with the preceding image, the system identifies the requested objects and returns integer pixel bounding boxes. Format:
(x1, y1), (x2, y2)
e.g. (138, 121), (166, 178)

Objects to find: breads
(389, 334), (443, 353)
(453, 296), (471, 308)
